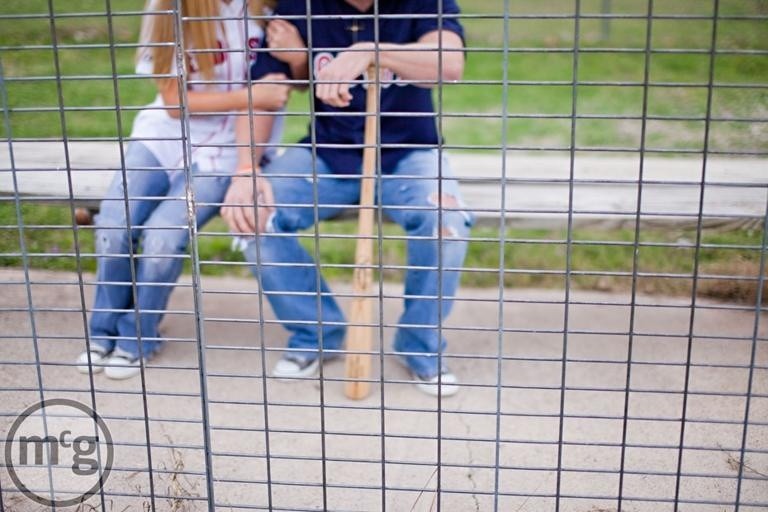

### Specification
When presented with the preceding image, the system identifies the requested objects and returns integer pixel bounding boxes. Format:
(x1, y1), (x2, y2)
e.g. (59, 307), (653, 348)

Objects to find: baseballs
(75, 207), (90, 225)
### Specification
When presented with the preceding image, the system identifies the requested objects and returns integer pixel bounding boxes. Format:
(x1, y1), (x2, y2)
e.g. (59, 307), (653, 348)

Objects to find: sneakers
(104, 336), (161, 381)
(394, 343), (460, 397)
(271, 333), (344, 382)
(74, 333), (112, 375)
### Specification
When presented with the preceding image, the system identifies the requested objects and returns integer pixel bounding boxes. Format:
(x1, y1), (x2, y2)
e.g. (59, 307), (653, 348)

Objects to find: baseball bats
(345, 64), (383, 400)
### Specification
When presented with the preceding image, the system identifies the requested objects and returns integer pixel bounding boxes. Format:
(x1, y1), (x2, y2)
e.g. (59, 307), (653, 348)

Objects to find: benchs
(0, 137), (768, 221)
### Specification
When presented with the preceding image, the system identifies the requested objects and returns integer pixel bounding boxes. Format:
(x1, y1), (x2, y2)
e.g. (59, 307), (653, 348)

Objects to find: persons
(74, 0), (313, 382)
(219, 0), (475, 398)
